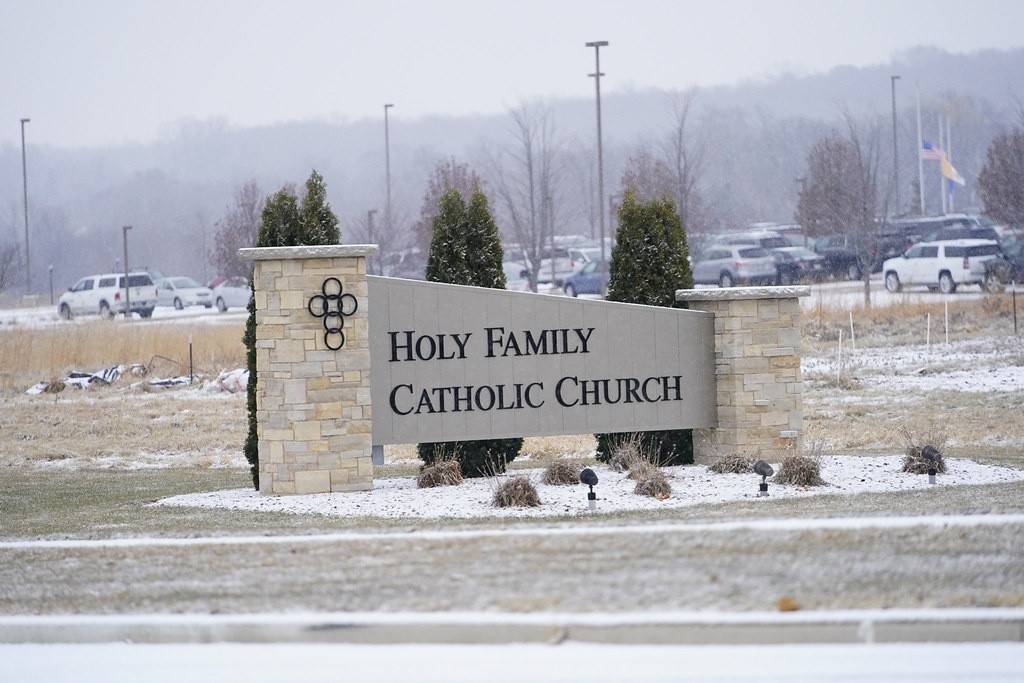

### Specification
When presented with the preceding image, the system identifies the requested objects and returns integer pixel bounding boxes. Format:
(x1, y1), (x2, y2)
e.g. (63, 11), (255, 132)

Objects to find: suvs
(58, 271), (158, 321)
(883, 240), (1004, 295)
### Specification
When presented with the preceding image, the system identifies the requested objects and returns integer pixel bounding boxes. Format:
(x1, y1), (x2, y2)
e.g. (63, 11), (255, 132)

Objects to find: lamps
(580, 469), (598, 510)
(922, 445), (942, 484)
(754, 461), (774, 497)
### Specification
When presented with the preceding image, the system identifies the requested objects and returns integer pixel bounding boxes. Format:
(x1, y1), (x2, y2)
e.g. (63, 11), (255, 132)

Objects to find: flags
(942, 158), (966, 185)
(922, 140), (944, 160)
(948, 179), (956, 192)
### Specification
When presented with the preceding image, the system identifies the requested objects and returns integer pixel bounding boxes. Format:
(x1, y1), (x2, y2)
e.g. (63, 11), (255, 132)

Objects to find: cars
(772, 246), (832, 287)
(691, 244), (779, 287)
(564, 258), (615, 296)
(872, 212), (1024, 285)
(691, 223), (865, 281)
(152, 276), (215, 309)
(500, 235), (620, 281)
(212, 275), (252, 312)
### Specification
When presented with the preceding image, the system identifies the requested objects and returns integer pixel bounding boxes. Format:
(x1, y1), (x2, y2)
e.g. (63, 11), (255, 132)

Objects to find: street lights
(584, 41), (615, 297)
(890, 76), (902, 220)
(384, 104), (393, 221)
(20, 118), (33, 296)
(123, 225), (133, 320)
(367, 210), (378, 244)
